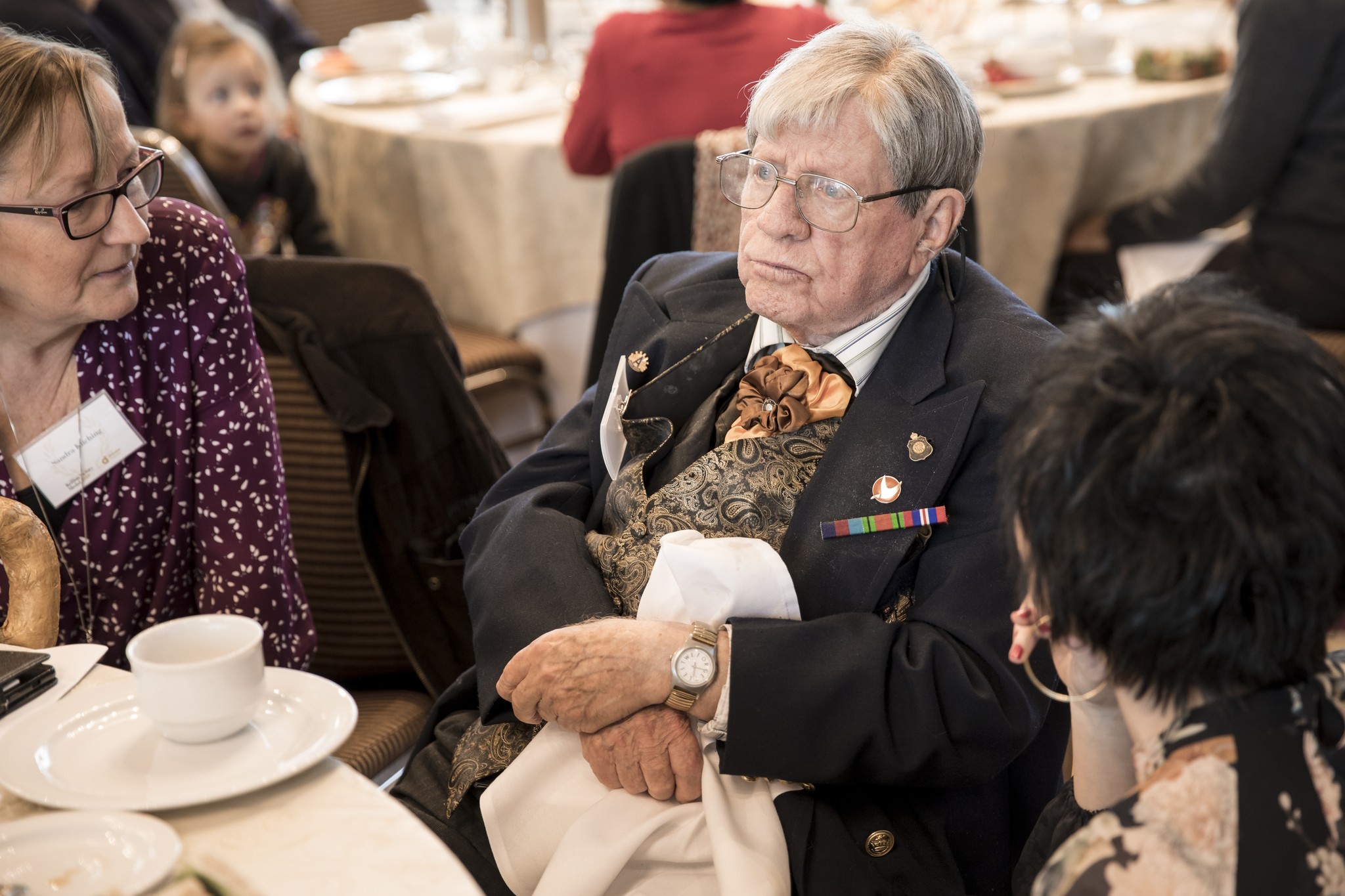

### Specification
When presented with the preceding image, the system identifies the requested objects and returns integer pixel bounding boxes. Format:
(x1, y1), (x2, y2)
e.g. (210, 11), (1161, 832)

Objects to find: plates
(299, 44), (466, 111)
(1, 812), (182, 896)
(0, 666), (359, 812)
(970, 63), (1084, 95)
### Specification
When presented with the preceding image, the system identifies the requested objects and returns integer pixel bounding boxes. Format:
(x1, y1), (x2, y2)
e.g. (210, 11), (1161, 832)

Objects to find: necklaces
(0, 389), (93, 644)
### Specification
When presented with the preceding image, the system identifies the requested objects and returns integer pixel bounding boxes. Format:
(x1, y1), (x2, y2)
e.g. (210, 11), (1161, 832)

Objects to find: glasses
(714, 149), (944, 233)
(0, 147), (165, 240)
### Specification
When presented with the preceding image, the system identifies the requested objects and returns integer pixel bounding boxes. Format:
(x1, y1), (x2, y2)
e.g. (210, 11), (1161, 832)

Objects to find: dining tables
(0, 643), (490, 896)
(296, 1), (1248, 371)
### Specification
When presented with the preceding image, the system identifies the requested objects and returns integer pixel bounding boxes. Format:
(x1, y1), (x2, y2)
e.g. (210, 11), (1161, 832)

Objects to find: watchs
(665, 621), (719, 711)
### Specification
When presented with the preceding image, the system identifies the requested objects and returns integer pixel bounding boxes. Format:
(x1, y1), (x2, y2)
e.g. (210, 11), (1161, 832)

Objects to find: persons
(1047, 1), (1344, 357)
(460, 20), (1069, 895)
(0, 34), (316, 677)
(0, 1), (349, 257)
(1008, 282), (1345, 896)
(563, 0), (839, 177)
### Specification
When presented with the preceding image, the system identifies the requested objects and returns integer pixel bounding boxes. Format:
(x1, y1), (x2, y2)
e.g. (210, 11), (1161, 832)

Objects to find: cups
(125, 613), (265, 744)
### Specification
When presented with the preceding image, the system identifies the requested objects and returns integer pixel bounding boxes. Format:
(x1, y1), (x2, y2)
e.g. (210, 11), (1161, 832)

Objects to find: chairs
(241, 250), (506, 780)
(130, 130), (560, 464)
